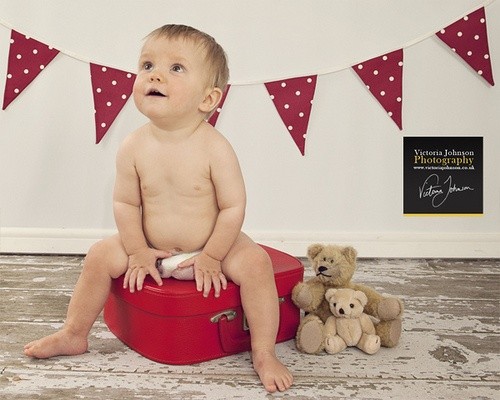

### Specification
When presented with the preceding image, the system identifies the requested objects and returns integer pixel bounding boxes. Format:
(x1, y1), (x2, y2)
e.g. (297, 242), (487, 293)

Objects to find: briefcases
(102, 243), (303, 367)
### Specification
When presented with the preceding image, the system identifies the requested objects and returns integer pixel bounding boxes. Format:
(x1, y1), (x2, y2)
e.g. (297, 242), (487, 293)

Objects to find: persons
(17, 23), (296, 395)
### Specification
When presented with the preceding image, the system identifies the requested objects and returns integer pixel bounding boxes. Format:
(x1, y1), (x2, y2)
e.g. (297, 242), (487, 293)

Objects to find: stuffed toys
(324, 287), (382, 354)
(290, 240), (403, 356)
